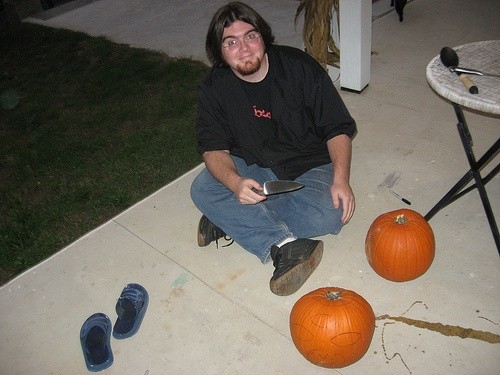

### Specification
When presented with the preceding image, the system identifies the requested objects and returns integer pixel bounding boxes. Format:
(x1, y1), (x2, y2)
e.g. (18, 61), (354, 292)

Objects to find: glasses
(221, 33), (263, 49)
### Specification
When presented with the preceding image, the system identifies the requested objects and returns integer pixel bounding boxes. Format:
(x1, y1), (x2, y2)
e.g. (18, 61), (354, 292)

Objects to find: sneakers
(197, 215), (234, 249)
(269, 238), (323, 297)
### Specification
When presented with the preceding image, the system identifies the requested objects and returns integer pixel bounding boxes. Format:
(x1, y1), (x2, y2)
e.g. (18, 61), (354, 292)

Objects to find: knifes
(253, 180), (304, 197)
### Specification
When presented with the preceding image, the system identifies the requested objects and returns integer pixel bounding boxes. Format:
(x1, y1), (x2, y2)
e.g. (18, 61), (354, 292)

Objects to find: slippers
(112, 283), (149, 340)
(80, 313), (114, 372)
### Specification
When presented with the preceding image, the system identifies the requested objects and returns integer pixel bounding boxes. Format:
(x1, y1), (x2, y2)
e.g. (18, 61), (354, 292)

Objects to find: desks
(423, 40), (500, 256)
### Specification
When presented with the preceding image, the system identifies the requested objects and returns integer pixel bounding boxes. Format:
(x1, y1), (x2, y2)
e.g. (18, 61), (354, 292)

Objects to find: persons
(190, 3), (357, 296)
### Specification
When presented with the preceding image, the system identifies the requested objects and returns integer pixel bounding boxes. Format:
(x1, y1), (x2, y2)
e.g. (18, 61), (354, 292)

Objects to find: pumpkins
(365, 208), (435, 282)
(289, 287), (375, 368)
